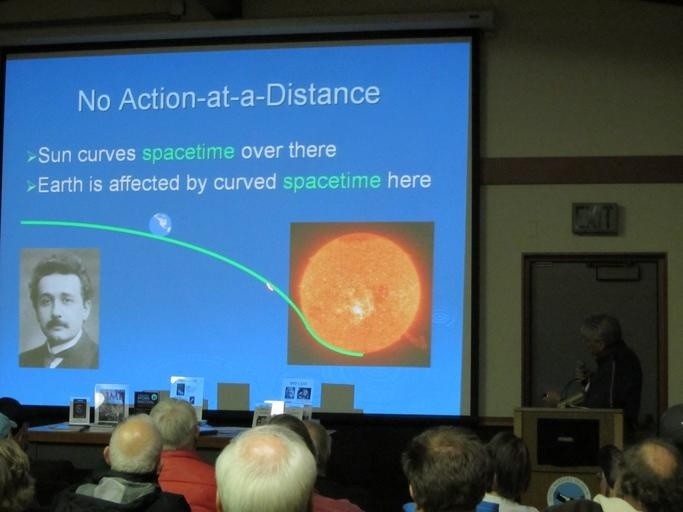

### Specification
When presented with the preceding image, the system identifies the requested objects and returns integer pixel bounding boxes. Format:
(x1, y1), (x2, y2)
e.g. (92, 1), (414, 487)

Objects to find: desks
(20, 423), (335, 467)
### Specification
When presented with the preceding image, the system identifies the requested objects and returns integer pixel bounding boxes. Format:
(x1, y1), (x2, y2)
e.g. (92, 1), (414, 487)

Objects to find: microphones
(576, 360), (584, 384)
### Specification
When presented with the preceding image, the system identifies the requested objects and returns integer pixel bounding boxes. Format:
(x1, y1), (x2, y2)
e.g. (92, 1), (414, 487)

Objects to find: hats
(0, 414), (16, 436)
(1, 397), (27, 433)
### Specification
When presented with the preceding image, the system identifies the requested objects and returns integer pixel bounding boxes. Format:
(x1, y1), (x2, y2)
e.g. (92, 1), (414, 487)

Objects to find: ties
(46, 348), (67, 367)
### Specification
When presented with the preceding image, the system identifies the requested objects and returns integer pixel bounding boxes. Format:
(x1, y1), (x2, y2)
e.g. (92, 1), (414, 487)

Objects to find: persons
(18, 254), (99, 369)
(542, 313), (643, 450)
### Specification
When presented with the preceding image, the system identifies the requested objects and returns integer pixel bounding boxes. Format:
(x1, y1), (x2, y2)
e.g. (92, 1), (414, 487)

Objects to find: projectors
(133, 389), (169, 410)
